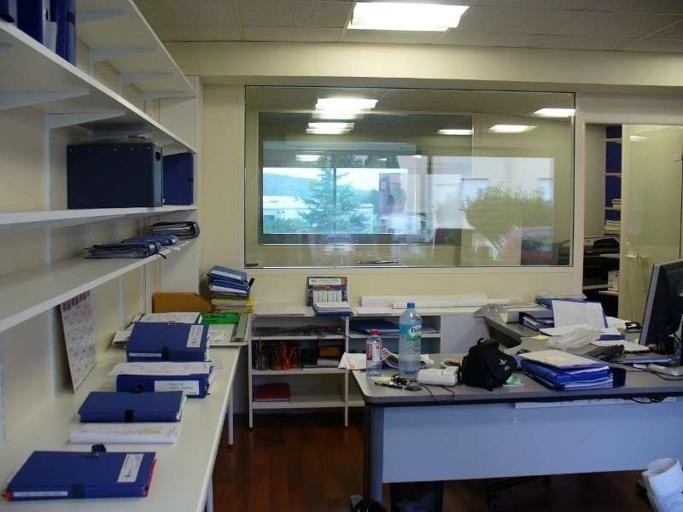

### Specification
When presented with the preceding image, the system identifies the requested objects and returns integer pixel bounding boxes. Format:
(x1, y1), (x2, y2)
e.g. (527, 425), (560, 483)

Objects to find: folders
(207, 266), (249, 294)
(84, 221), (200, 258)
(77, 321), (214, 422)
(519, 298), (625, 340)
(4, 444), (156, 501)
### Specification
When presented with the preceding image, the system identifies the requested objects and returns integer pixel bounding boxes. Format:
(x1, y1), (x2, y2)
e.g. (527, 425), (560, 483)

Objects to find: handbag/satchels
(459, 339), (518, 389)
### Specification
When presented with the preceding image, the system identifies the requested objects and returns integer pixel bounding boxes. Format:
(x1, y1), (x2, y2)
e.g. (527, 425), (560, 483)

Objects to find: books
(299, 340), (344, 369)
(251, 382), (292, 403)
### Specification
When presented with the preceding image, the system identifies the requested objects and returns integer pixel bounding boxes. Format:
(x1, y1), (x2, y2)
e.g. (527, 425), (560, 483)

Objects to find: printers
(553, 233), (621, 286)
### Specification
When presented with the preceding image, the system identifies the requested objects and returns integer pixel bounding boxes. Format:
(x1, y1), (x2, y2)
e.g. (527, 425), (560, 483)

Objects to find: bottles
(366, 329), (382, 381)
(398, 301), (422, 379)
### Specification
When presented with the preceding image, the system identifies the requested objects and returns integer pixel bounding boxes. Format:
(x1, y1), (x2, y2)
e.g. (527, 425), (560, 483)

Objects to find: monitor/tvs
(637, 259), (683, 365)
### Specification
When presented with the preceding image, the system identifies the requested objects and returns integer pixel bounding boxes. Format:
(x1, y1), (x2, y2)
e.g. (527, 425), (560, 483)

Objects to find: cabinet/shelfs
(248, 303), (493, 431)
(601, 126), (622, 296)
(0, 0), (200, 337)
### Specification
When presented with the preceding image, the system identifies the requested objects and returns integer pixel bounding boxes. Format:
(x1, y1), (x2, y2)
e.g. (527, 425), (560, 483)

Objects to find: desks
(351, 316), (681, 510)
(1, 343), (241, 512)
